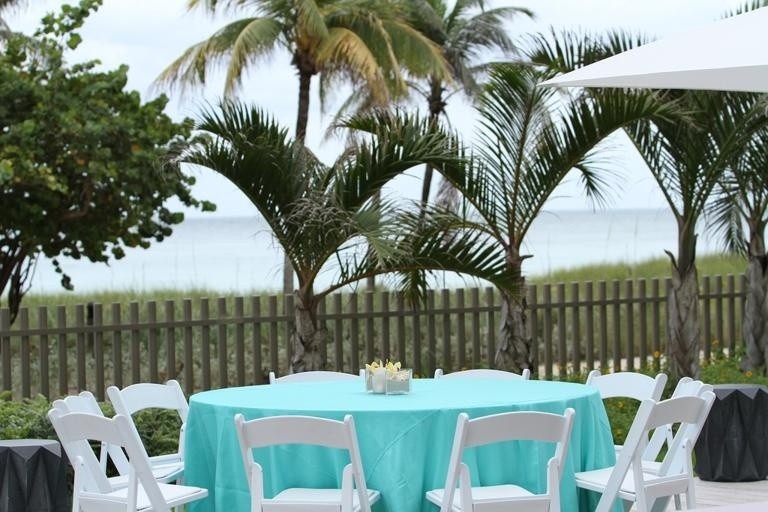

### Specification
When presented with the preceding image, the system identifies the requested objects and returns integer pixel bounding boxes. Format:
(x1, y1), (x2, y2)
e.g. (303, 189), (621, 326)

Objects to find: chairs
(435, 367), (532, 382)
(235, 412), (382, 512)
(268, 370), (367, 387)
(575, 370), (716, 512)
(426, 408), (575, 512)
(45, 379), (210, 512)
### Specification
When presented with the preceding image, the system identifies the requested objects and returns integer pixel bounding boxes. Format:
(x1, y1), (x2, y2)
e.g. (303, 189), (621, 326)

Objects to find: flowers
(366, 359), (413, 394)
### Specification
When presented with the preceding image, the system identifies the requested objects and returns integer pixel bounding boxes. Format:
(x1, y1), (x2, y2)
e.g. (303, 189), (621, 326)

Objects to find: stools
(0, 438), (68, 512)
(692, 382), (768, 482)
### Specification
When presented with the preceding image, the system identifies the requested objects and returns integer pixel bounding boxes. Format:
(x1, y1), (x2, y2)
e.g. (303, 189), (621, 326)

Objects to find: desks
(183, 378), (626, 512)
